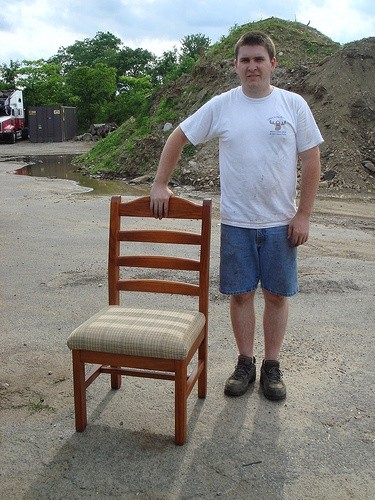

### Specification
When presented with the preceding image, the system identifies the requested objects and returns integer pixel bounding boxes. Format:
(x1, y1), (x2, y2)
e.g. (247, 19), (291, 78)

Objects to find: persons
(149, 31), (325, 400)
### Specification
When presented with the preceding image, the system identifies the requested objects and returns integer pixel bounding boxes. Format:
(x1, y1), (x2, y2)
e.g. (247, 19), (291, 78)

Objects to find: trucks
(0, 88), (28, 144)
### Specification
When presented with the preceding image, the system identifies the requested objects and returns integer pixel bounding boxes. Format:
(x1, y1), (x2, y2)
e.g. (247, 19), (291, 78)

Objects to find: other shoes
(224, 354), (256, 396)
(259, 359), (286, 400)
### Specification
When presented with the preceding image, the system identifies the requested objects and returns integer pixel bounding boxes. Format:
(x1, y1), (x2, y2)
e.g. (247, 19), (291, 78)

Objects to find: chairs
(65, 194), (214, 446)
